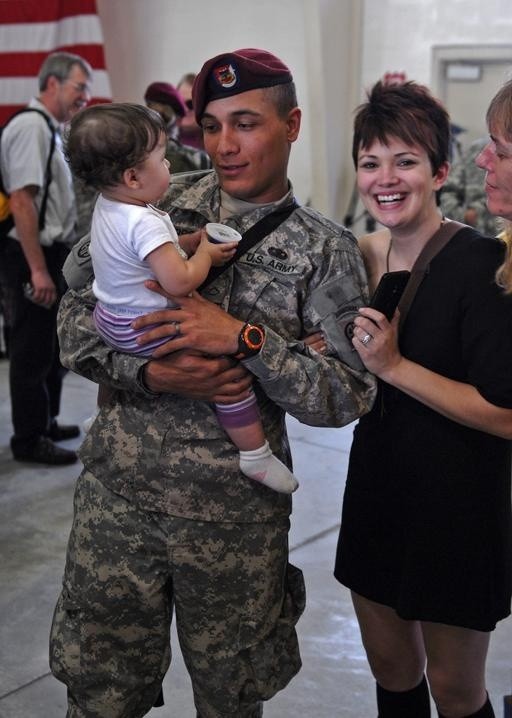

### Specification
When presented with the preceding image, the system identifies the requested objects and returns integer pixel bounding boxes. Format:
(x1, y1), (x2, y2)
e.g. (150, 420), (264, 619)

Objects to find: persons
(440, 136), (505, 241)
(175, 71), (205, 153)
(63, 102), (299, 499)
(60, 81), (213, 249)
(46, 48), (378, 717)
(300, 78), (512, 718)
(475, 78), (511, 295)
(0, 51), (94, 466)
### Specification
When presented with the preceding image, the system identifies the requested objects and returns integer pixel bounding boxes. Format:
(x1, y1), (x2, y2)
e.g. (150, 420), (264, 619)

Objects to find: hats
(191, 47), (293, 131)
(143, 82), (189, 119)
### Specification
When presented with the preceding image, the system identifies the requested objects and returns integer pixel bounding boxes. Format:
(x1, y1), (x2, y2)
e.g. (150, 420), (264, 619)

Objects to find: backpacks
(0, 107), (56, 242)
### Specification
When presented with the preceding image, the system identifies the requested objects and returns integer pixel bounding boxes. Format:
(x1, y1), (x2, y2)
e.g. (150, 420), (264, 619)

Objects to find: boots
(435, 688), (495, 718)
(374, 673), (433, 718)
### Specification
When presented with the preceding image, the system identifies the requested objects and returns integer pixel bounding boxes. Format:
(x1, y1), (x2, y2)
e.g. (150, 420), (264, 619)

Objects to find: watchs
(231, 321), (265, 361)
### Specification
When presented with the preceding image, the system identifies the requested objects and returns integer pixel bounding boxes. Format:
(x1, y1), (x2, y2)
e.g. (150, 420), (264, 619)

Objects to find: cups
(204, 222), (242, 247)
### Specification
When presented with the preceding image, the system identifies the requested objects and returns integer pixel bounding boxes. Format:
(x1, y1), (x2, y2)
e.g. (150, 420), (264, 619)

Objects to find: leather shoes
(10, 435), (78, 467)
(40, 421), (82, 443)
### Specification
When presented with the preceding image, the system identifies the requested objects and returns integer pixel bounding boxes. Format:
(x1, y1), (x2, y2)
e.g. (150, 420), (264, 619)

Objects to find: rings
(175, 322), (180, 336)
(362, 334), (371, 345)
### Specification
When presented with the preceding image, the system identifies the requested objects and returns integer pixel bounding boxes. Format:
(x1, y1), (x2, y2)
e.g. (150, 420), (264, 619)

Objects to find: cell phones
(365, 271), (408, 324)
